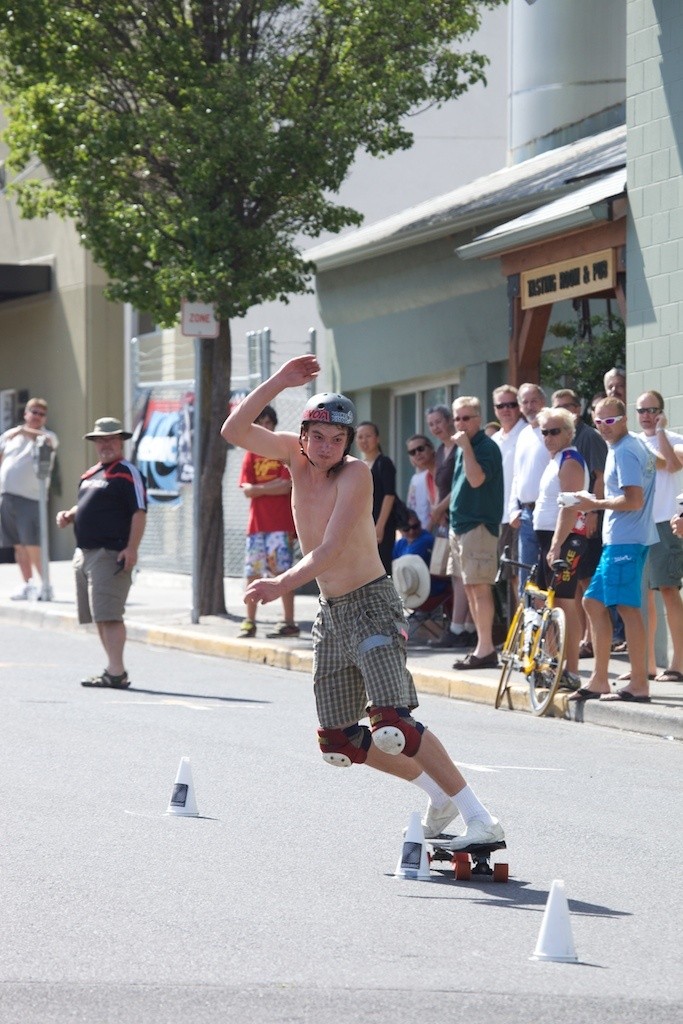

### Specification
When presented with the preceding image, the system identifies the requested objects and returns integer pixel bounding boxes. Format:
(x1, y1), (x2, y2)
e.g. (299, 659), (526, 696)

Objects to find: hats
(391, 554), (430, 608)
(83, 417), (133, 441)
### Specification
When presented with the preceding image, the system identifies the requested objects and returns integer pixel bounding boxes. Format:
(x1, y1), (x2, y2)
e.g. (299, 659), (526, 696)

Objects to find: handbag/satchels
(393, 500), (410, 530)
(429, 531), (451, 577)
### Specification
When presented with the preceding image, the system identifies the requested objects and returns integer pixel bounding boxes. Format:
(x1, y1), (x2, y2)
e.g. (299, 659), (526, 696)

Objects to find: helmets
(301, 392), (357, 437)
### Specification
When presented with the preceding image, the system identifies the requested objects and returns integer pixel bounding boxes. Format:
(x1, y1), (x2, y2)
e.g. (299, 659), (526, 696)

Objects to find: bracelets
(655, 429), (665, 432)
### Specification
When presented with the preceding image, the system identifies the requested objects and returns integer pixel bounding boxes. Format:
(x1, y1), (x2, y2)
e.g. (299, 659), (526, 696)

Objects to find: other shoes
(238, 620), (256, 637)
(454, 650), (498, 669)
(265, 621), (301, 637)
(39, 587), (54, 600)
(11, 585), (37, 600)
(427, 629), (470, 647)
(578, 640), (594, 658)
(528, 669), (582, 691)
(613, 641), (627, 652)
(470, 631), (477, 646)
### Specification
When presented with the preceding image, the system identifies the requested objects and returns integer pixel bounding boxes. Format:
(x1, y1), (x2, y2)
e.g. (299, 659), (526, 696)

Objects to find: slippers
(568, 666), (683, 703)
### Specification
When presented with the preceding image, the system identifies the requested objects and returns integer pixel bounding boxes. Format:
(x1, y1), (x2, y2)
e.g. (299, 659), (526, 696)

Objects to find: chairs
(403, 589), (452, 639)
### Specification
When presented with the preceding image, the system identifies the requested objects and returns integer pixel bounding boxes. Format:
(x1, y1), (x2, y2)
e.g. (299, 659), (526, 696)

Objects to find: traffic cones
(164, 755), (202, 818)
(387, 810), (436, 882)
(525, 878), (581, 964)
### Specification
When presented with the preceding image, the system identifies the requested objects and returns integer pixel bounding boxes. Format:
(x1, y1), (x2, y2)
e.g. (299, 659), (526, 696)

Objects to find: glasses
(409, 444), (428, 456)
(494, 401), (518, 409)
(28, 409), (46, 417)
(400, 521), (421, 532)
(636, 407), (660, 414)
(453, 414), (480, 422)
(590, 406), (595, 411)
(593, 415), (623, 426)
(541, 427), (569, 436)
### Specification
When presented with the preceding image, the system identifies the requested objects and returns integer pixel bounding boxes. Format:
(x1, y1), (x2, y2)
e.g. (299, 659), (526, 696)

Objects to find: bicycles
(494, 546), (571, 718)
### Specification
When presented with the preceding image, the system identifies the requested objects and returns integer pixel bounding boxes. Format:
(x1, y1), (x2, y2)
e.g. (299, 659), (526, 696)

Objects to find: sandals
(81, 670), (131, 687)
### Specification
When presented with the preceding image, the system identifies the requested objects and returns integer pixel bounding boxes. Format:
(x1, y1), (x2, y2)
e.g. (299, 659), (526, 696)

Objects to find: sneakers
(404, 799), (460, 838)
(450, 817), (504, 850)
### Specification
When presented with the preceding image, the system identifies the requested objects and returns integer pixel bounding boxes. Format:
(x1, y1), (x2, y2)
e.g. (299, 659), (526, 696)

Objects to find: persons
(354, 370), (683, 702)
(236, 406), (300, 639)
(220, 354), (503, 851)
(55, 417), (148, 687)
(0, 398), (60, 602)
